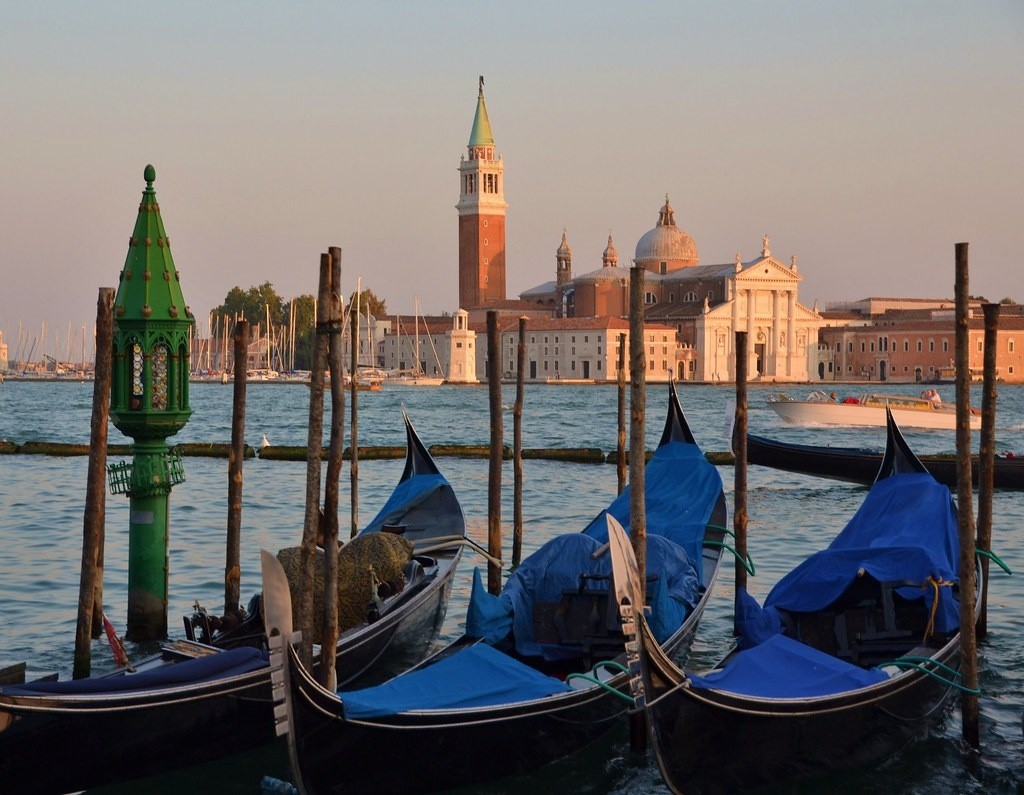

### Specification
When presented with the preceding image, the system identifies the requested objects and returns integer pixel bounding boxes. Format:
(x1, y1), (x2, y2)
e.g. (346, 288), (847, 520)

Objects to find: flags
(103, 617), (137, 673)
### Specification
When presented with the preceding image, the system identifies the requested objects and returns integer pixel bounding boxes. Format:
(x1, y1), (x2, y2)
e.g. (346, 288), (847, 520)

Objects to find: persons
(828, 393), (839, 403)
(920, 389), (941, 408)
(763, 234), (769, 250)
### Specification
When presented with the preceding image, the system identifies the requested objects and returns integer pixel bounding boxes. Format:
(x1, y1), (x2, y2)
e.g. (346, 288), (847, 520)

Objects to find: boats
(259, 369), (728, 794)
(604, 398), (985, 795)
(765, 388), (982, 430)
(0, 402), (467, 795)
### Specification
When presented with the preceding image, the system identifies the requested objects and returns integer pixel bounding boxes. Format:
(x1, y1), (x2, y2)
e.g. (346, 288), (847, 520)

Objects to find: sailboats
(0, 276), (388, 392)
(381, 294), (445, 386)
